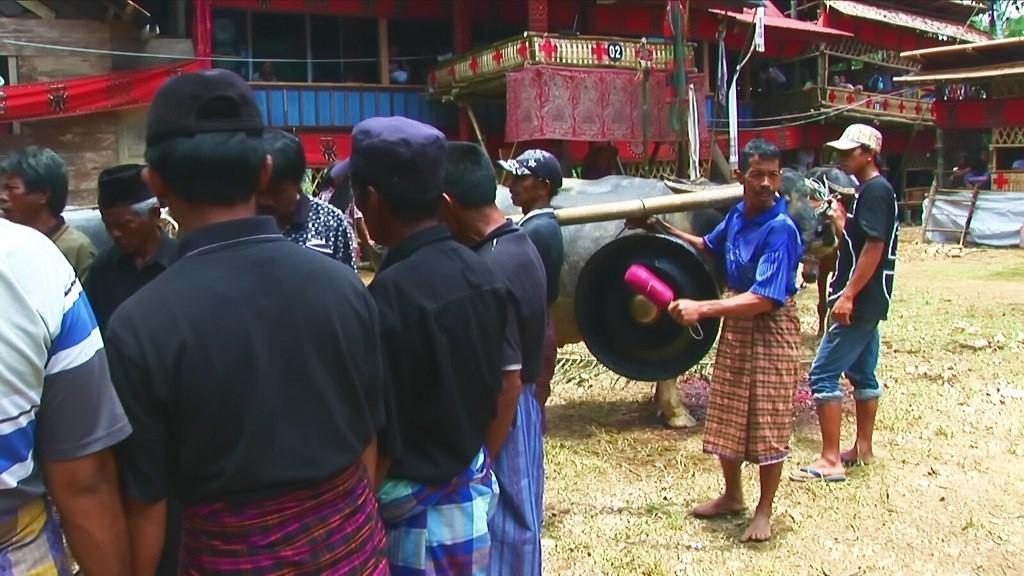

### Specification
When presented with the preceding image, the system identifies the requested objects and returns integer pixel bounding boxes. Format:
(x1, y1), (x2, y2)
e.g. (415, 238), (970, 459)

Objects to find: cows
(495, 175), (838, 426)
(780, 166), (859, 338)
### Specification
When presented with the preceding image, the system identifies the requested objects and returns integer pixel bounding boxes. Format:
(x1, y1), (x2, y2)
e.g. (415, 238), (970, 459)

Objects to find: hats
(496, 149), (562, 189)
(825, 124), (883, 153)
(96, 163), (152, 209)
(330, 116), (447, 188)
(146, 68), (265, 147)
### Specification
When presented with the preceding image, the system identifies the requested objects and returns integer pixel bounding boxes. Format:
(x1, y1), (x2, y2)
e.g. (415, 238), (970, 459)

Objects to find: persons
(0, 67), (564, 576)
(251, 56), (284, 81)
(788, 123), (899, 484)
(804, 75), (863, 92)
(749, 63), (783, 128)
(387, 45), (408, 84)
(947, 146), (1024, 190)
(624, 138), (802, 542)
(581, 147), (618, 180)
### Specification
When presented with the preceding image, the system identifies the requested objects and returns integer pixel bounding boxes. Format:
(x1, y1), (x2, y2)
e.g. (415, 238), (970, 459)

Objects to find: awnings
(709, 9), (854, 37)
(893, 36), (1024, 84)
(824, 0), (997, 44)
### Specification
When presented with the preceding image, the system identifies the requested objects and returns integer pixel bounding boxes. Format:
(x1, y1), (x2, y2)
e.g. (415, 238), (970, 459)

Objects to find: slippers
(841, 456), (875, 465)
(789, 467), (845, 482)
